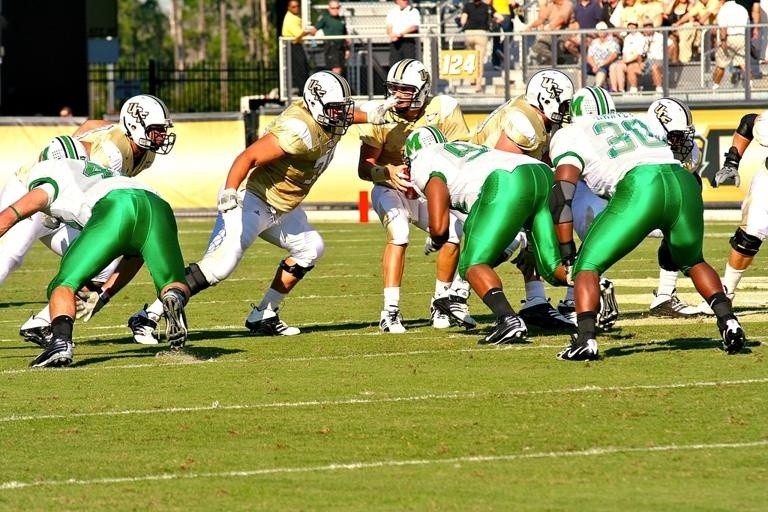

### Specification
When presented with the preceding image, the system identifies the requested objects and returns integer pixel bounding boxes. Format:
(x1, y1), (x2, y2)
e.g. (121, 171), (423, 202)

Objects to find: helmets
(648, 97), (696, 162)
(40, 136), (89, 162)
(120, 94), (177, 156)
(386, 58), (432, 119)
(401, 126), (448, 167)
(302, 70), (355, 136)
(525, 70), (575, 129)
(568, 86), (616, 118)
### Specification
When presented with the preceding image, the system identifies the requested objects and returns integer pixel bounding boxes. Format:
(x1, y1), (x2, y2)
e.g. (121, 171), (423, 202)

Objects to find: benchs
(305, 0), (768, 86)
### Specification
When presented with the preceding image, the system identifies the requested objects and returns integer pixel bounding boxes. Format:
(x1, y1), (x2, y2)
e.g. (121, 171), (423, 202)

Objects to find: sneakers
(161, 287), (189, 352)
(554, 333), (599, 361)
(127, 303), (161, 346)
(246, 302), (302, 336)
(378, 305), (407, 333)
(717, 314), (747, 355)
(697, 285), (736, 317)
(649, 288), (702, 318)
(430, 277), (620, 346)
(19, 314), (54, 350)
(28, 337), (74, 367)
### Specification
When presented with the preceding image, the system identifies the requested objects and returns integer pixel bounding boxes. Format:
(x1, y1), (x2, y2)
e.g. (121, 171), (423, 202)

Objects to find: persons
(0, 95), (176, 349)
(128, 72), (399, 346)
(1, 137), (188, 368)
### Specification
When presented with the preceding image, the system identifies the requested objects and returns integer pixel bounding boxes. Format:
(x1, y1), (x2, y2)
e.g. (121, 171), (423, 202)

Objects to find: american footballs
(402, 168), (419, 199)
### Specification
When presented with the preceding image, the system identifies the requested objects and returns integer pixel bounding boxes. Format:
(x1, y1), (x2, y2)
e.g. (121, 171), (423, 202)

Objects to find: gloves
(75, 287), (110, 324)
(511, 252), (541, 280)
(710, 147), (741, 188)
(366, 95), (399, 125)
(217, 188), (244, 213)
(424, 234), (449, 255)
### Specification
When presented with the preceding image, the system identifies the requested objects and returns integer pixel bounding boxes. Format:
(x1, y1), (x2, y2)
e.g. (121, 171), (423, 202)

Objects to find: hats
(596, 21), (608, 31)
